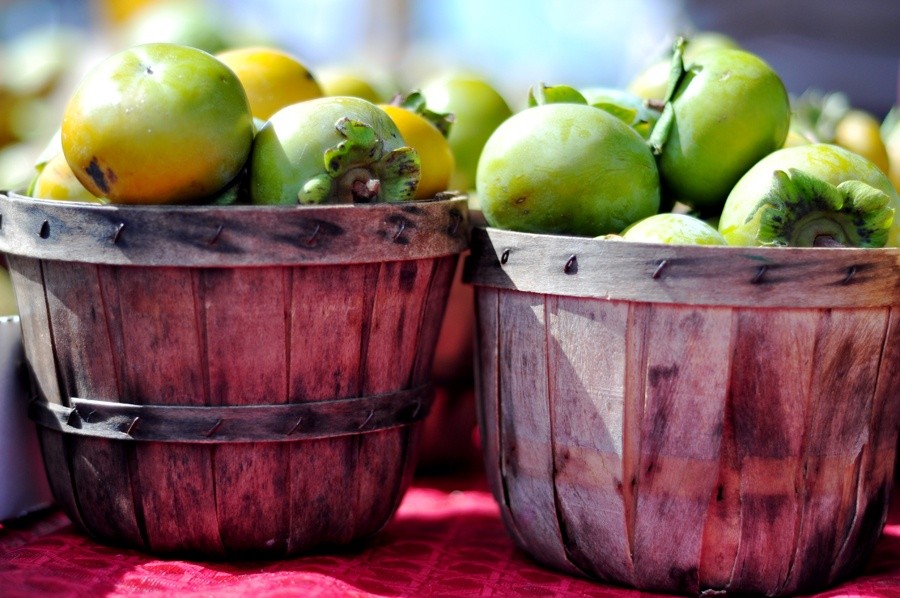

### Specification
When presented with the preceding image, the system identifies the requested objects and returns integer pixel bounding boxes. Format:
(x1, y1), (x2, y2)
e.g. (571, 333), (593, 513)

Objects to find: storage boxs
(0, 316), (53, 524)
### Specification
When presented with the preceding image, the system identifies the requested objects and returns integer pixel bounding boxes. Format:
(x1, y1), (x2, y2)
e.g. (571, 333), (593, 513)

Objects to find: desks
(0, 428), (900, 598)
